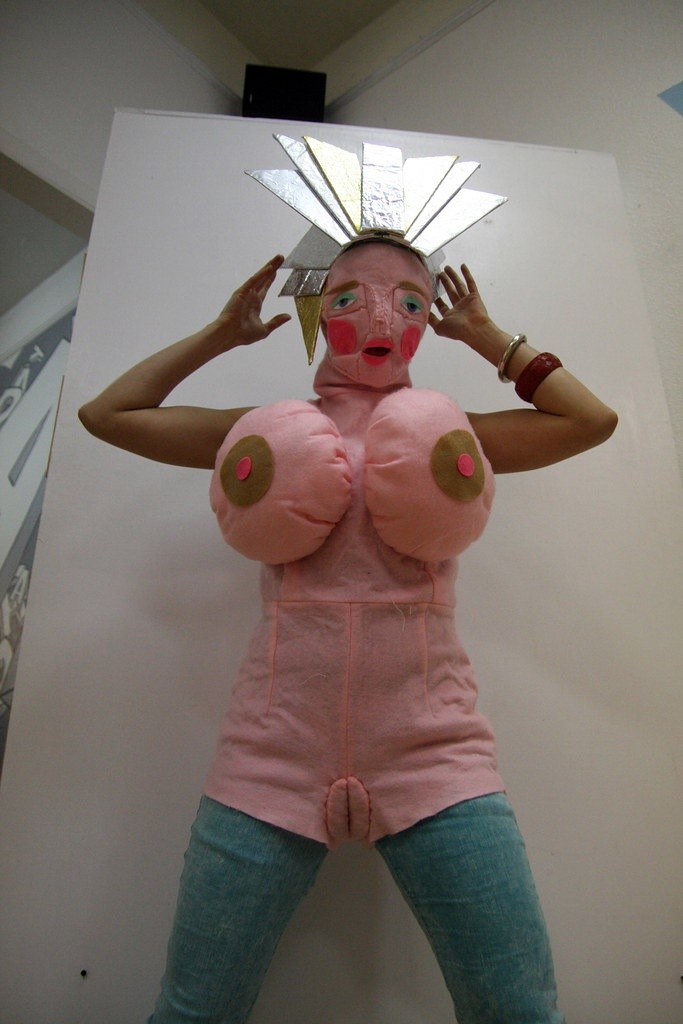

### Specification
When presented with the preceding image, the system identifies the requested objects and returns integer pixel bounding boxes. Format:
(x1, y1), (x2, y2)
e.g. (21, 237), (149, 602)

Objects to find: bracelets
(515, 352), (563, 402)
(498, 333), (527, 384)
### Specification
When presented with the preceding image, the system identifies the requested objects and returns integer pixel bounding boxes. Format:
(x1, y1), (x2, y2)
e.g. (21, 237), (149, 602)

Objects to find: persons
(78, 236), (620, 1023)
